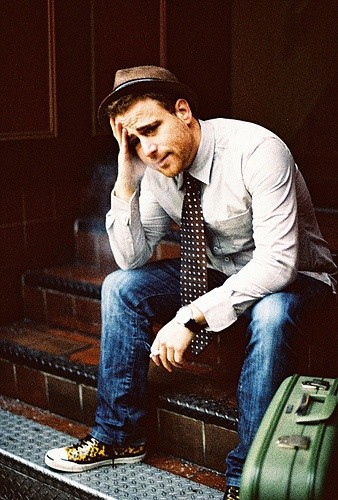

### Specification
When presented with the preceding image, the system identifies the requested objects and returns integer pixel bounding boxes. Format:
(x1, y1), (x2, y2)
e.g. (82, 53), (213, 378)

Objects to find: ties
(180, 173), (213, 356)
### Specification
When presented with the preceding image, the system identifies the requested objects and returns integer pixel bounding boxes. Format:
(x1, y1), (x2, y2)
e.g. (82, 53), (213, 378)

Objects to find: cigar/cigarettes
(149, 348), (163, 359)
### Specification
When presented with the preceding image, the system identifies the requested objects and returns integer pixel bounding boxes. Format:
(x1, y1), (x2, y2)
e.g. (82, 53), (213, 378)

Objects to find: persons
(44, 65), (338, 500)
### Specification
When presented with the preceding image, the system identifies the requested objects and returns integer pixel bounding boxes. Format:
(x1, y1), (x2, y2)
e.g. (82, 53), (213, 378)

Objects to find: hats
(97, 66), (201, 133)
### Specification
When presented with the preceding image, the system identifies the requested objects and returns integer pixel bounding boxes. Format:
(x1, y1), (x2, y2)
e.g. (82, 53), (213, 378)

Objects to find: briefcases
(239, 374), (338, 500)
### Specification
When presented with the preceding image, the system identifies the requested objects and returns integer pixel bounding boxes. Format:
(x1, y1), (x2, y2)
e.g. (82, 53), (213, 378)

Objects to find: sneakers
(45, 434), (147, 473)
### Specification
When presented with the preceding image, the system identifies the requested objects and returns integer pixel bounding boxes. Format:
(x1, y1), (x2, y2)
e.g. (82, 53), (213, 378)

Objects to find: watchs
(175, 306), (206, 335)
